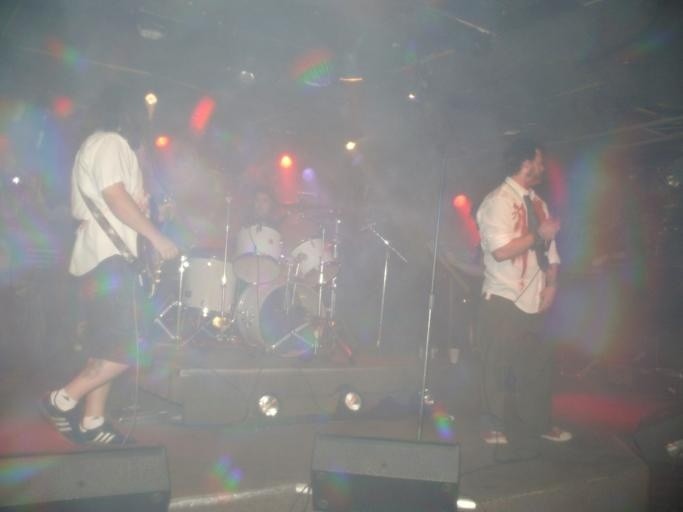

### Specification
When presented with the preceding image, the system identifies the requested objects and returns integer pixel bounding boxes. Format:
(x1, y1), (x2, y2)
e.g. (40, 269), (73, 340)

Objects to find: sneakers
(537, 422), (573, 441)
(37, 387), (85, 445)
(77, 419), (132, 445)
(480, 428), (508, 444)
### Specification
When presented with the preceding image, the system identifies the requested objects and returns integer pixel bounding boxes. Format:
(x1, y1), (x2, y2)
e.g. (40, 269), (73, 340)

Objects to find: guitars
(137, 185), (167, 299)
(425, 241), (479, 307)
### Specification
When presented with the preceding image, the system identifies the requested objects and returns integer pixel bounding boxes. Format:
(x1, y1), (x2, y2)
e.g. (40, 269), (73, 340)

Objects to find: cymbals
(317, 206), (353, 227)
(285, 200), (319, 211)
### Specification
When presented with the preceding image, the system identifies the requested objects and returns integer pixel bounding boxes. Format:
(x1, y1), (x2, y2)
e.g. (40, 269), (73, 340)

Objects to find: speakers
(635, 406), (683, 463)
(0, 441), (172, 512)
(308, 431), (463, 512)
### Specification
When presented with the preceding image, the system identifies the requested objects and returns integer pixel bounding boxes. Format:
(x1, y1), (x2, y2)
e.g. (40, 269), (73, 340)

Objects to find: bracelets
(533, 229), (545, 246)
(545, 278), (557, 287)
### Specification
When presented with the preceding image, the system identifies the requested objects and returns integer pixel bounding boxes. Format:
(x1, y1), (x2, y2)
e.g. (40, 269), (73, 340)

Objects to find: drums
(238, 225), (284, 284)
(288, 237), (340, 278)
(237, 280), (326, 358)
(181, 258), (235, 314)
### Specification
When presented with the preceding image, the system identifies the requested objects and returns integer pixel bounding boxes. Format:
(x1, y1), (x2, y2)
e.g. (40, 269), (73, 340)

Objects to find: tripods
(151, 202), (362, 365)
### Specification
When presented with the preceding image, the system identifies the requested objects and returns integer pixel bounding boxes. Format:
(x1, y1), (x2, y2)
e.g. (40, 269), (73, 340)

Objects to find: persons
(34, 101), (180, 447)
(474, 137), (573, 445)
(242, 186), (289, 233)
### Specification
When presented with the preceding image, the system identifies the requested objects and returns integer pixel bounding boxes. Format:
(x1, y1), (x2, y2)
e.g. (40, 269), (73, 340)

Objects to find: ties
(526, 195), (545, 270)
